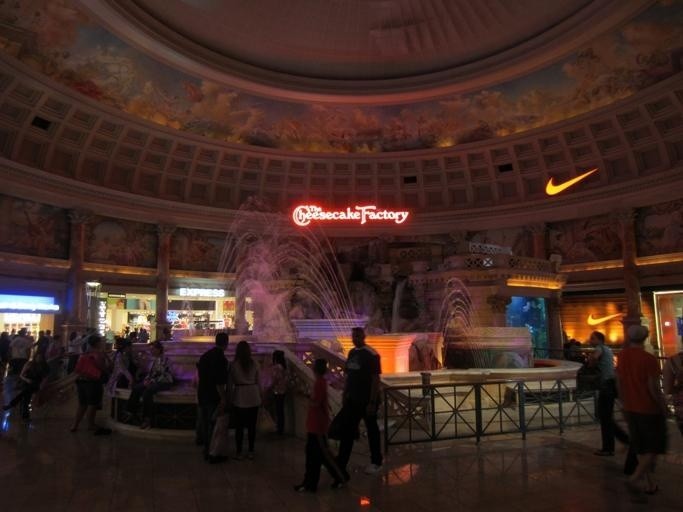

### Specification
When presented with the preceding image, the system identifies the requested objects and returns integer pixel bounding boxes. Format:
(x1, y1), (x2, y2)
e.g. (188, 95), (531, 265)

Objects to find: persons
(574, 341), (585, 360)
(291, 357), (350, 492)
(584, 330), (636, 456)
(226, 339), (263, 462)
(569, 338), (575, 361)
(268, 350), (290, 436)
(192, 332), (229, 448)
(0, 316), (176, 433)
(331, 326), (384, 470)
(660, 343), (683, 441)
(612, 323), (669, 494)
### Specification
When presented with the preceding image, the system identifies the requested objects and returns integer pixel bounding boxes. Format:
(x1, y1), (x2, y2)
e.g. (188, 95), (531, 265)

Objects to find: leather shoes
(594, 449), (615, 457)
(292, 483), (316, 493)
(331, 477), (350, 490)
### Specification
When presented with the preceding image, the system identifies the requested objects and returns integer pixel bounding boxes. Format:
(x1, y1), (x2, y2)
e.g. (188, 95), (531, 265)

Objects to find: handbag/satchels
(575, 365), (600, 392)
(255, 407), (273, 433)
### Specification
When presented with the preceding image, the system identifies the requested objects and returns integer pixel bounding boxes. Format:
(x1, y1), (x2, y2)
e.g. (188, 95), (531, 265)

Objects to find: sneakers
(365, 464), (382, 474)
